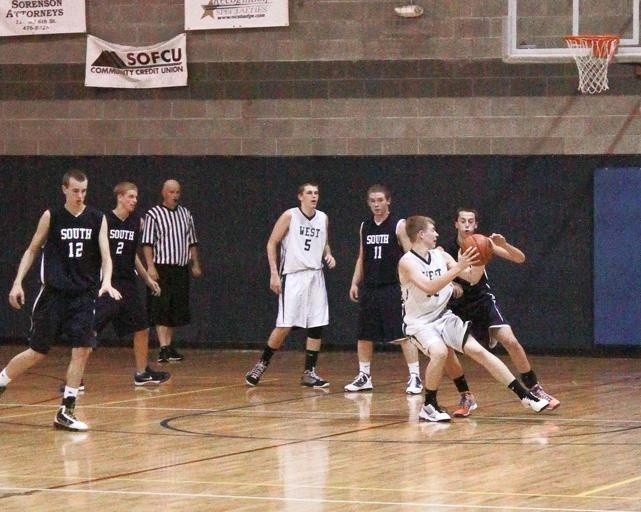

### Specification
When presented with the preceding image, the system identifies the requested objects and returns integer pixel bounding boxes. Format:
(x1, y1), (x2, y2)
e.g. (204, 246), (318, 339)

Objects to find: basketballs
(461, 233), (493, 265)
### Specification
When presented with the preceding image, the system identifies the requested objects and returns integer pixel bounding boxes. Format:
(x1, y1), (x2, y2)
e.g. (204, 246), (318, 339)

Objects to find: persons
(246, 386), (330, 512)
(396, 216), (550, 423)
(444, 208), (561, 419)
(60, 182), (171, 392)
(344, 184), (423, 395)
(1, 170), (124, 432)
(139, 179), (205, 363)
(245, 181), (336, 388)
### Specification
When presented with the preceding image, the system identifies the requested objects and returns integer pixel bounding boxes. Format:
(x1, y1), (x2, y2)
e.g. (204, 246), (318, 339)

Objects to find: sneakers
(244, 361), (266, 386)
(134, 366), (170, 387)
(300, 368), (330, 387)
(405, 372), (424, 394)
(453, 391), (478, 417)
(530, 382), (561, 410)
(158, 345), (183, 362)
(344, 371), (374, 392)
(54, 405), (89, 432)
(419, 404), (452, 423)
(520, 391), (549, 413)
(60, 383), (86, 392)
